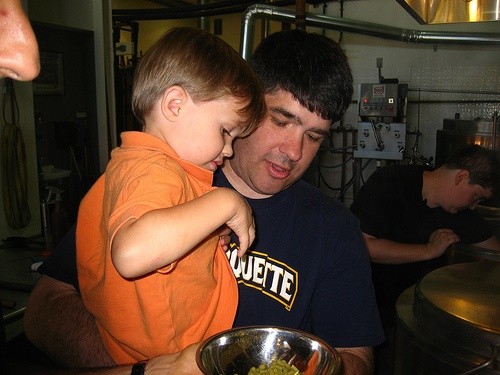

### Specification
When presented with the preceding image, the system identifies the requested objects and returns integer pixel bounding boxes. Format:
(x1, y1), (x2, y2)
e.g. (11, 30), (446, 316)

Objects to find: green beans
(248, 360), (302, 375)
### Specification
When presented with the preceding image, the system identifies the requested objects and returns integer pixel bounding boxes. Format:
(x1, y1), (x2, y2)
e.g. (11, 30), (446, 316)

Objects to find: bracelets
(130, 358), (149, 375)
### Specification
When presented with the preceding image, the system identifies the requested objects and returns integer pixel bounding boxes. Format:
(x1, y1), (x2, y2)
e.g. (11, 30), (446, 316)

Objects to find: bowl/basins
(195, 325), (341, 375)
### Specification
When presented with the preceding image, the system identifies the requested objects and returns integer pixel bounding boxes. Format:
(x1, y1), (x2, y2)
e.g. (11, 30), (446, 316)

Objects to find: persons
(0, 0), (208, 375)
(23, 30), (387, 375)
(347, 143), (500, 375)
(75, 28), (265, 366)
